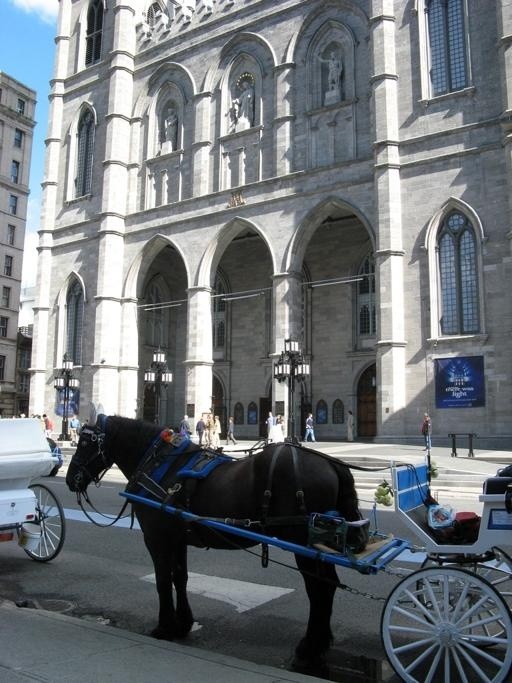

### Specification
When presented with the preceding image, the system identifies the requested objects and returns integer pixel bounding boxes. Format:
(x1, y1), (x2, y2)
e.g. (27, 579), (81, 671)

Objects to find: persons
(265, 412), (285, 444)
(420, 414), (432, 451)
(196, 414), (220, 444)
(225, 417), (237, 445)
(304, 413), (314, 441)
(67, 415), (79, 446)
(33, 413), (51, 438)
(347, 410), (354, 441)
(180, 415), (190, 438)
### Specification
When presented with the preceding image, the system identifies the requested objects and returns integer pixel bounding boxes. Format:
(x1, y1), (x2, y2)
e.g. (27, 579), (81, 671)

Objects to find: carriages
(65, 413), (512, 682)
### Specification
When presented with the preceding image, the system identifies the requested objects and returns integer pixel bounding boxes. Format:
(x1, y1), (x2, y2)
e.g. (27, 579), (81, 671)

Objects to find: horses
(65, 400), (364, 678)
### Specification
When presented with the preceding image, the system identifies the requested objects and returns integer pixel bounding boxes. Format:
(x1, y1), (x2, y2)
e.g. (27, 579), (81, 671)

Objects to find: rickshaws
(0, 413), (65, 561)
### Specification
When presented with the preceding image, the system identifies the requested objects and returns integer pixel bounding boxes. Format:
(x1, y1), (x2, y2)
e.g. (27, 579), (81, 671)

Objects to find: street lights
(143, 344), (173, 420)
(52, 350), (80, 440)
(271, 332), (311, 447)
